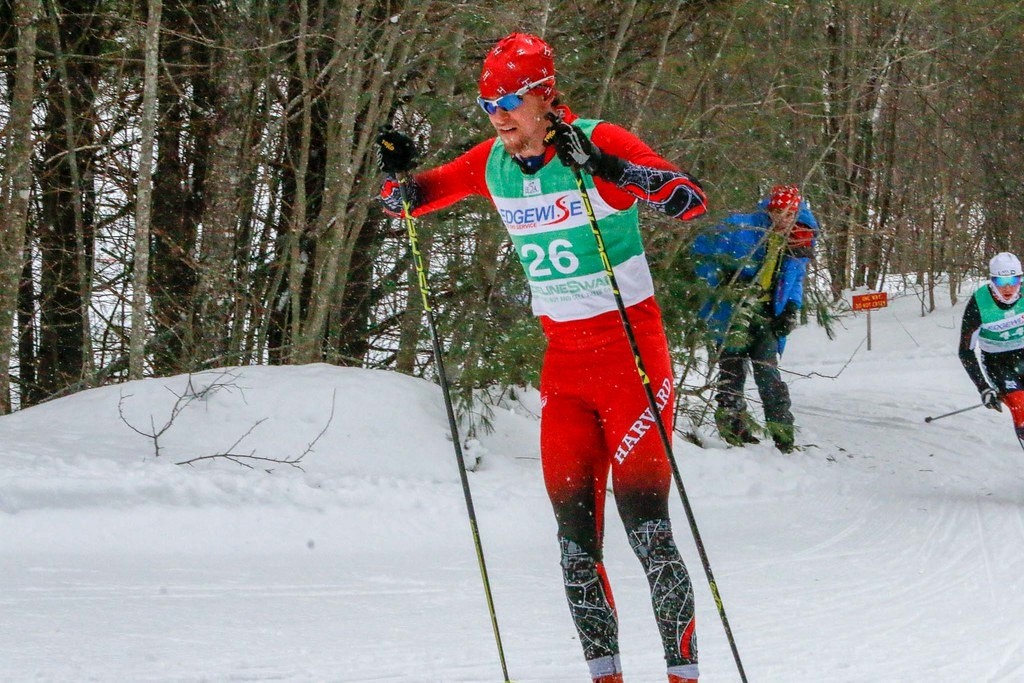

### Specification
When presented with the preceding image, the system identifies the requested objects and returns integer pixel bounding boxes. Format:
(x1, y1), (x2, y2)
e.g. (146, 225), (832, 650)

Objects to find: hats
(769, 184), (800, 211)
(479, 30), (554, 99)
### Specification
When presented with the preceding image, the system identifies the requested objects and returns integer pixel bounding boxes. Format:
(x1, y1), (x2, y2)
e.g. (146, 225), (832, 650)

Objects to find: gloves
(542, 121), (605, 177)
(982, 387), (1005, 413)
(374, 131), (419, 172)
(773, 303), (798, 338)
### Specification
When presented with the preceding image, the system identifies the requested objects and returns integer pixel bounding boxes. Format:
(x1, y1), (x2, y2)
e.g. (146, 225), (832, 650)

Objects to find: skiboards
(682, 430), (855, 463)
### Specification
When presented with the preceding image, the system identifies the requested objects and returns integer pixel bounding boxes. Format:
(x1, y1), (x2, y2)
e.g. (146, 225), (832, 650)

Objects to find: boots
(762, 382), (794, 453)
(714, 394), (761, 445)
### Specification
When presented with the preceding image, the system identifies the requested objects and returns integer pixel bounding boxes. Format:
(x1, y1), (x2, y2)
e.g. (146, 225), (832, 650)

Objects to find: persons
(379, 34), (709, 683)
(692, 183), (819, 453)
(958, 251), (1024, 448)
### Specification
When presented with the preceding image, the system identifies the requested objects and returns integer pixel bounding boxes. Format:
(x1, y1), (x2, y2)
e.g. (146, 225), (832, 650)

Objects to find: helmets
(988, 251), (1023, 305)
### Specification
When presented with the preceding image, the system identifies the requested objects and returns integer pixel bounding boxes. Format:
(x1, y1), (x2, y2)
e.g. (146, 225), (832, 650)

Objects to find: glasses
(476, 75), (554, 115)
(990, 276), (1021, 287)
